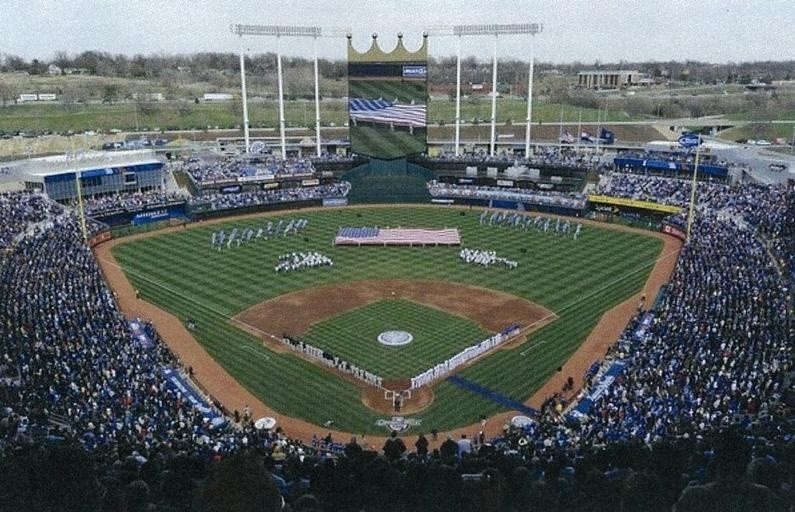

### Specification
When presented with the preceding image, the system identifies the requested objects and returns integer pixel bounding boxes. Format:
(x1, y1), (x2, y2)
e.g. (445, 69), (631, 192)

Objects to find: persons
(3, 142), (794, 512)
(390, 121), (398, 130)
(352, 115), (359, 126)
(409, 122), (416, 136)
(377, 96), (385, 101)
(392, 96), (404, 104)
(411, 97), (419, 105)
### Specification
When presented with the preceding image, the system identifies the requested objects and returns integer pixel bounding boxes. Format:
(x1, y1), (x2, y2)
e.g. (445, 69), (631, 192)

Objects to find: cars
(743, 137), (791, 145)
(0, 121), (350, 139)
(438, 117), (494, 125)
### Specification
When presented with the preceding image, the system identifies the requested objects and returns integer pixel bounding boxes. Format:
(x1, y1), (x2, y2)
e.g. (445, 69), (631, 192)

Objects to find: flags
(350, 96), (427, 129)
